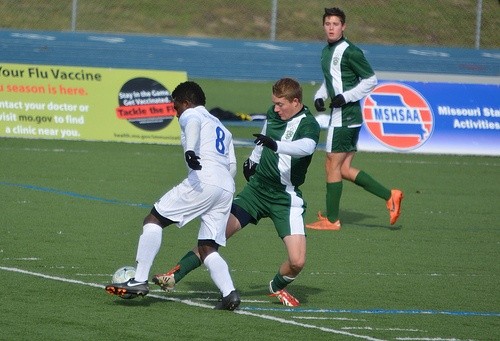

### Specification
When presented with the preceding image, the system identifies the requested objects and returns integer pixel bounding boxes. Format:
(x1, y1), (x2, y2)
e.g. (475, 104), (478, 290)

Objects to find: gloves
(243, 159), (258, 182)
(185, 151), (202, 170)
(329, 94), (346, 108)
(315, 99), (326, 112)
(252, 133), (278, 152)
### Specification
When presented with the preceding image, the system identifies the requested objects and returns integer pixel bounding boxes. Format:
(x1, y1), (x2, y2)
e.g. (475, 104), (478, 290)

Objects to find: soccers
(112, 266), (136, 286)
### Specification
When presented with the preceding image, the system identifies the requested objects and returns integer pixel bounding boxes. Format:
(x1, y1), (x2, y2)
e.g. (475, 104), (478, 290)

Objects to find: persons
(306, 7), (403, 230)
(153, 77), (321, 308)
(106, 80), (241, 310)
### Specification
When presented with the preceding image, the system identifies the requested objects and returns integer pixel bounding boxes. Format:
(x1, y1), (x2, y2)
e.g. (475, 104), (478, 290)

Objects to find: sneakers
(105, 279), (149, 298)
(214, 290), (240, 312)
(152, 272), (176, 293)
(305, 211), (342, 231)
(267, 280), (299, 308)
(386, 189), (405, 225)
